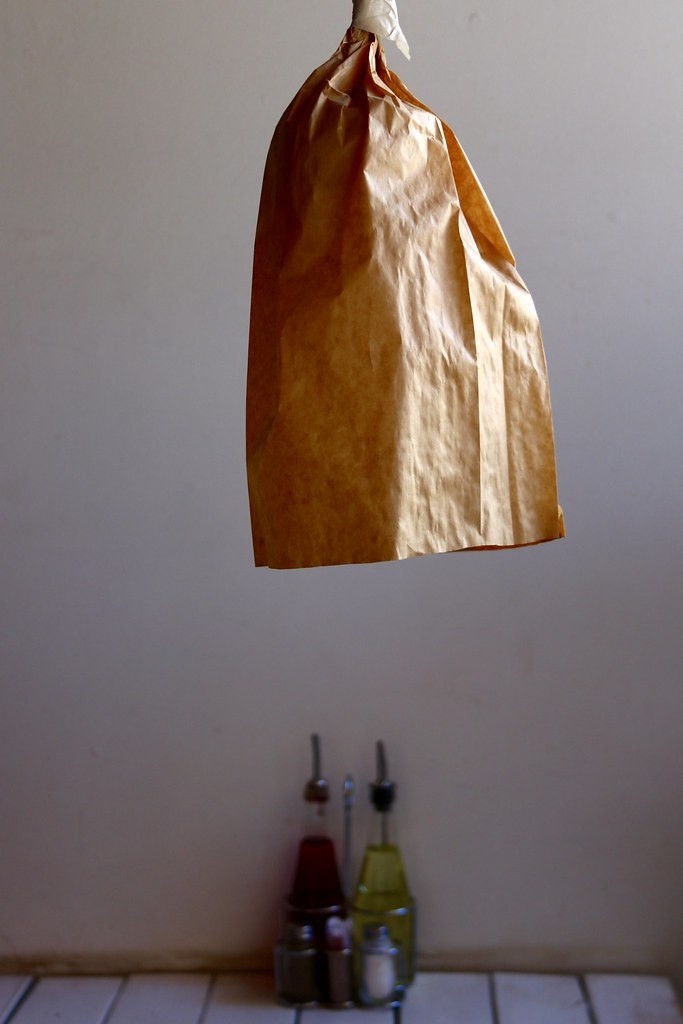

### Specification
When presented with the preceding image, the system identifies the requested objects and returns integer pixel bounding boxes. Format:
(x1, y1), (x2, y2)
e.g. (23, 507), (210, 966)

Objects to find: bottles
(313, 921), (353, 1008)
(281, 733), (345, 934)
(346, 739), (417, 988)
(275, 921), (318, 1008)
(357, 923), (405, 1006)
